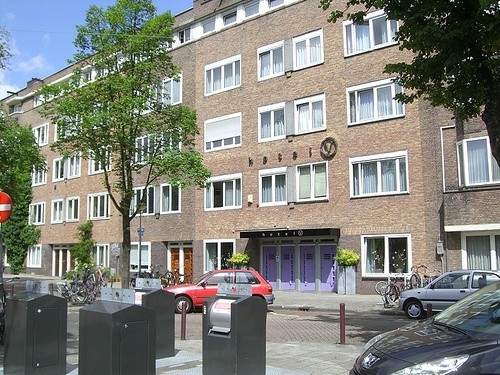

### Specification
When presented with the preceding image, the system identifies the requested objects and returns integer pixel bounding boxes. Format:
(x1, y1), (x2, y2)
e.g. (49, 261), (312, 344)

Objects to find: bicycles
(84, 264), (107, 287)
(130, 264), (191, 290)
(0, 277), (21, 344)
(375, 264), (452, 308)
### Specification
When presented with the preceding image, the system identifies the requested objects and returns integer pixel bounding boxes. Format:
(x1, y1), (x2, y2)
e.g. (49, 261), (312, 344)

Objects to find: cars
(396, 270), (500, 321)
(349, 280), (500, 375)
(162, 270), (276, 314)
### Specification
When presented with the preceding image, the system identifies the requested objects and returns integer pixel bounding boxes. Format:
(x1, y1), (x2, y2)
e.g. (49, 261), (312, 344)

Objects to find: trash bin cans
(135, 288), (175, 359)
(202, 294), (266, 375)
(78, 300), (156, 375)
(2, 291), (67, 375)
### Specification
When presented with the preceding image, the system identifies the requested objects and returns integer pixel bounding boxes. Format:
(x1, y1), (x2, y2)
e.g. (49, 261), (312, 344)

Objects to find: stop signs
(0, 192), (13, 223)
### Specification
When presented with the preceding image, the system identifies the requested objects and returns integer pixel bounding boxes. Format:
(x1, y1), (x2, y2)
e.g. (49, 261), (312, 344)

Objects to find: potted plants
(392, 248), (407, 273)
(371, 250), (383, 273)
(333, 246), (360, 295)
(226, 252), (255, 270)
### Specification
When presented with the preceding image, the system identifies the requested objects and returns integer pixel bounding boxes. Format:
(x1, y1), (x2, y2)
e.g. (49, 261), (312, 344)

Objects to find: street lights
(136, 201), (145, 277)
